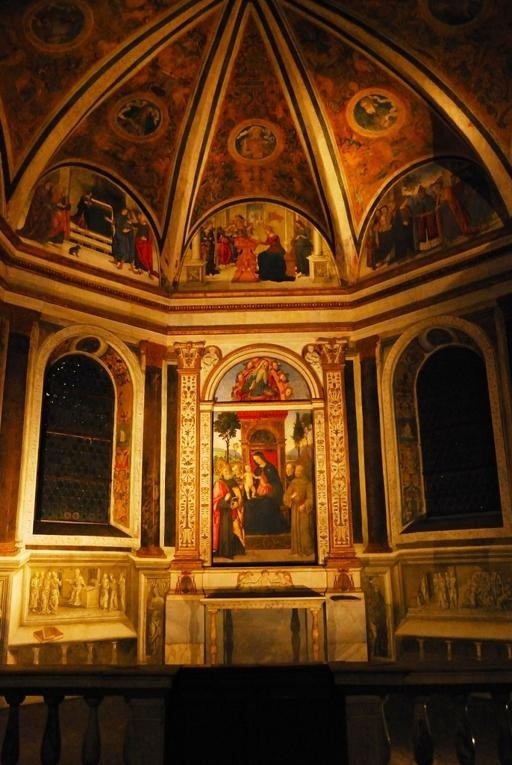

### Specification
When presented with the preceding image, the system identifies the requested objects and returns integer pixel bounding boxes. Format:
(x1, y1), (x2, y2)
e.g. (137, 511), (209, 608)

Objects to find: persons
(213, 451), (314, 560)
(241, 360), (275, 401)
